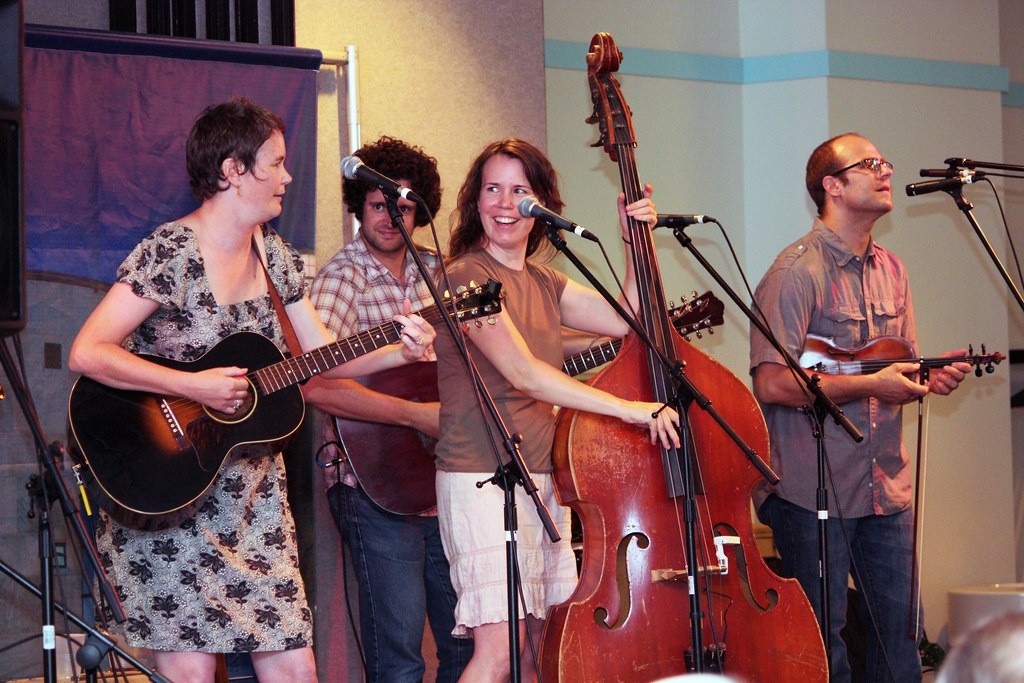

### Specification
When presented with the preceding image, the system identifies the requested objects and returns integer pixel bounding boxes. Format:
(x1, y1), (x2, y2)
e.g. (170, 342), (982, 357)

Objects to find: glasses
(821, 157), (893, 192)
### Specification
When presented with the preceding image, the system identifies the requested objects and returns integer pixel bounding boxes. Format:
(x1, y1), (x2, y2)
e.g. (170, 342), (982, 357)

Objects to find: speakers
(0, 117), (26, 337)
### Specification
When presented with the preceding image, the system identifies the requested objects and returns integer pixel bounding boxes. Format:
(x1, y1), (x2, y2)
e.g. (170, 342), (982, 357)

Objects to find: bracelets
(622, 236), (631, 244)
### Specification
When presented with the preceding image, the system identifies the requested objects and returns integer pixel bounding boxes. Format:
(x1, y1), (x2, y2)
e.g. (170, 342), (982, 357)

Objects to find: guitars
(67, 276), (505, 537)
(328, 290), (724, 517)
(792, 329), (1007, 406)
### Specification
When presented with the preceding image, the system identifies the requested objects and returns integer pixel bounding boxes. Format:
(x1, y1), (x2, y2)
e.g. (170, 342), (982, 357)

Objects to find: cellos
(534, 32), (831, 683)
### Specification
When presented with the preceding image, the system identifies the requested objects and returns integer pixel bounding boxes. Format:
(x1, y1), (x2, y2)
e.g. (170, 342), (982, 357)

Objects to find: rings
(416, 337), (424, 344)
(234, 400), (240, 409)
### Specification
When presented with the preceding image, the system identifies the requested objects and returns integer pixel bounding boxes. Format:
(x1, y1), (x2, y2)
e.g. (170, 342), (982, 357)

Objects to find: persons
(936, 609), (1024, 683)
(307, 137), (615, 683)
(436, 139), (683, 683)
(749, 132), (971, 683)
(67, 99), (435, 683)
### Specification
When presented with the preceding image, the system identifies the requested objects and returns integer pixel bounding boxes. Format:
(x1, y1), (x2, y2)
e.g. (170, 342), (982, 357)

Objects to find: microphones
(919, 167), (986, 177)
(517, 195), (599, 242)
(906, 175), (983, 195)
(652, 213), (715, 228)
(341, 157), (424, 206)
